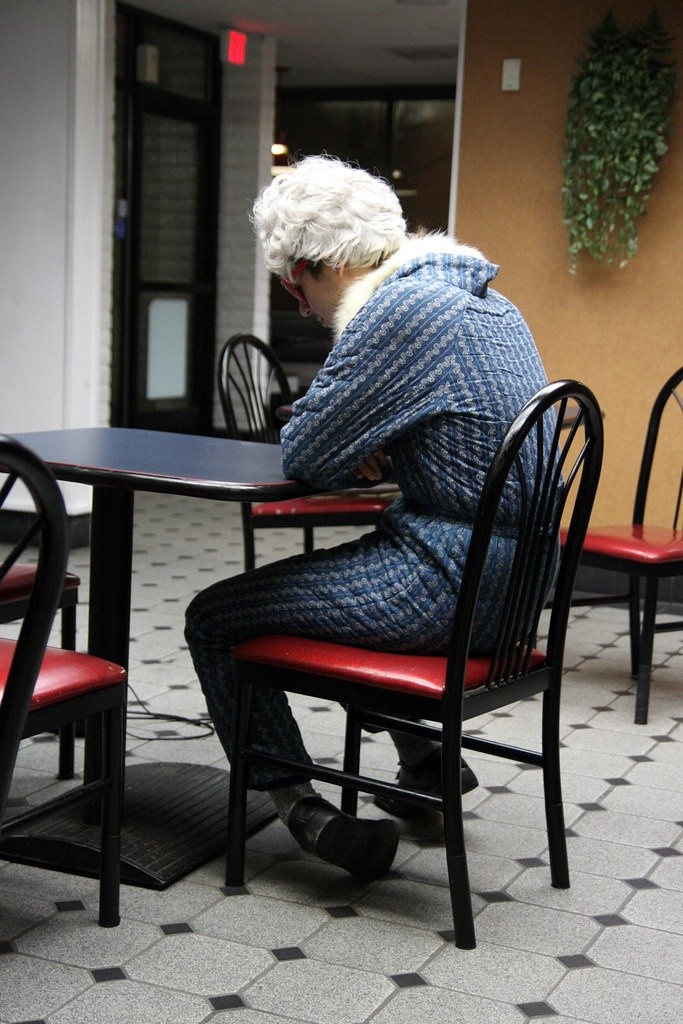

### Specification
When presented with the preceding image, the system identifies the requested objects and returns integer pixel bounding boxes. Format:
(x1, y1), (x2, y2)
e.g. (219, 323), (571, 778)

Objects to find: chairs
(557, 366), (683, 725)
(0, 561), (80, 779)
(217, 333), (398, 573)
(225, 379), (605, 951)
(0, 432), (125, 928)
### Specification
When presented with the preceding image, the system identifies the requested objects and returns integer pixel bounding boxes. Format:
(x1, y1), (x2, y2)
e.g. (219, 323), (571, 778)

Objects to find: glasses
(281, 259), (309, 305)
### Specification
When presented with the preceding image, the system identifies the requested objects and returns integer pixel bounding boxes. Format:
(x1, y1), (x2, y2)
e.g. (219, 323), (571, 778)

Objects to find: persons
(183, 154), (565, 880)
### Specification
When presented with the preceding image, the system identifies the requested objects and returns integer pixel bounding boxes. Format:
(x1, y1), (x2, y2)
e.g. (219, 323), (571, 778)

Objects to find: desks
(0, 428), (390, 891)
(276, 405), (606, 430)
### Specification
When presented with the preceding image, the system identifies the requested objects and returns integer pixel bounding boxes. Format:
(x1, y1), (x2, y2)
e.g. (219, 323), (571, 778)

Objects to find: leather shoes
(374, 723), (479, 821)
(286, 795), (402, 884)
